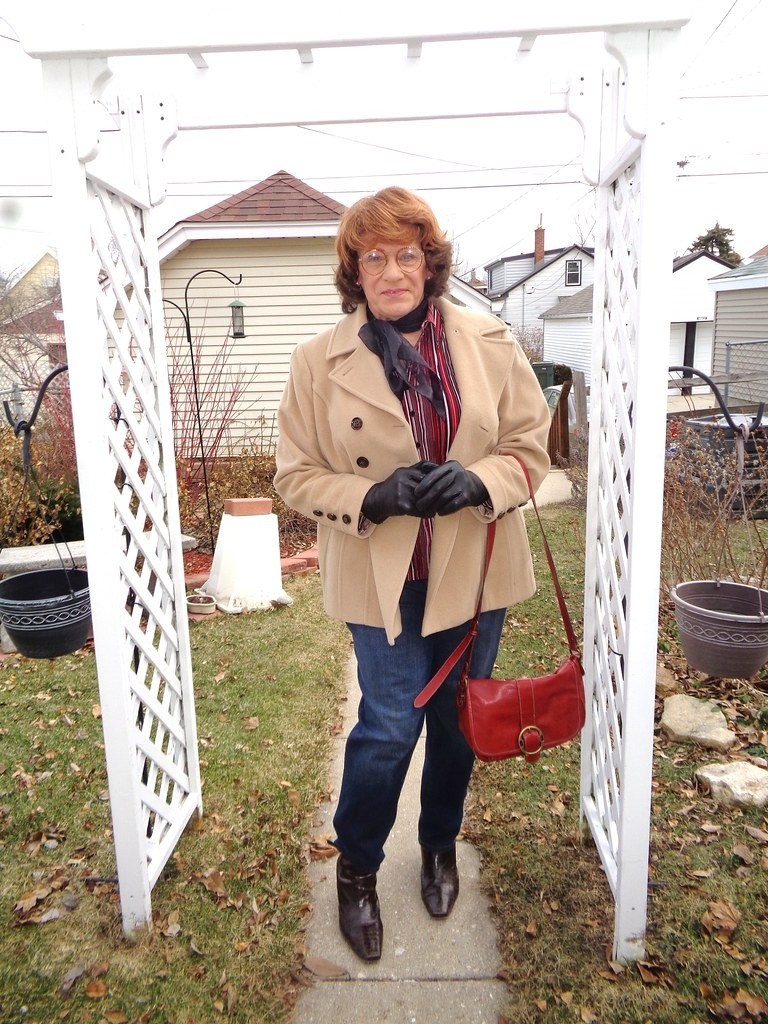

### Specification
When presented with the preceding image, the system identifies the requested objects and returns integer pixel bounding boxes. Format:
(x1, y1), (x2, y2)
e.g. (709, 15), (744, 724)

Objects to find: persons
(274, 186), (552, 965)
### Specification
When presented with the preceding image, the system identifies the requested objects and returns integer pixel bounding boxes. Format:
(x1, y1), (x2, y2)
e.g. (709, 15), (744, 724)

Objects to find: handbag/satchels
(456, 655), (586, 763)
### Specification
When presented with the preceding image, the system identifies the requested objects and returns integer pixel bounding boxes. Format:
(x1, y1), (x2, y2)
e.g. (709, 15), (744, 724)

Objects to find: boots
(327, 839), (383, 961)
(421, 841), (460, 917)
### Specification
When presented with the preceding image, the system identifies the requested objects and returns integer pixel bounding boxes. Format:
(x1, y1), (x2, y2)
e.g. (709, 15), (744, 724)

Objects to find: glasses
(357, 246), (426, 276)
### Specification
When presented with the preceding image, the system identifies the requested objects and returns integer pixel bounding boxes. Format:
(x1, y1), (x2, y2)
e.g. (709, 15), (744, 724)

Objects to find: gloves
(361, 460), (428, 524)
(415, 459), (489, 516)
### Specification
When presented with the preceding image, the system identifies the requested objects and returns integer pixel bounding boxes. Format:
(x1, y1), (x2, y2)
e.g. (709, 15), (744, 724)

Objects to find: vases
(0, 567), (90, 659)
(670, 581), (768, 680)
